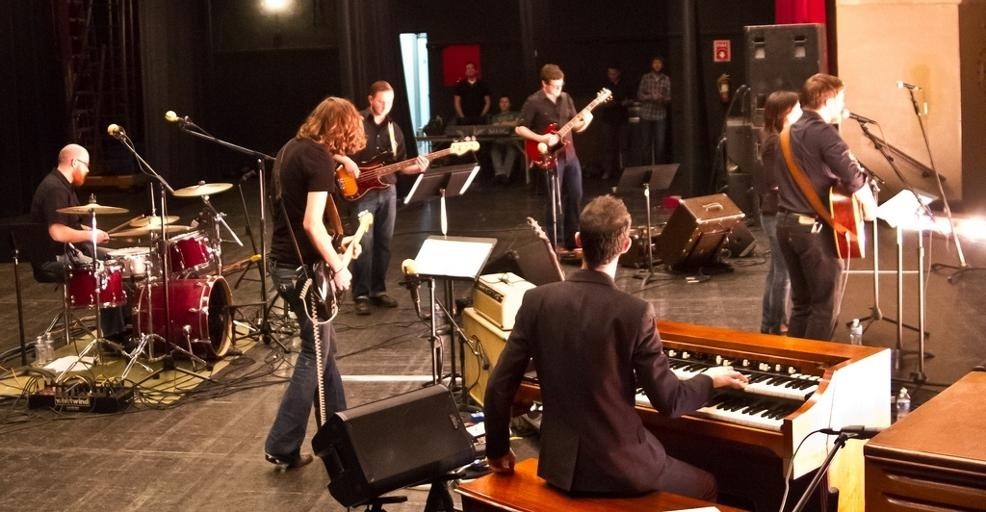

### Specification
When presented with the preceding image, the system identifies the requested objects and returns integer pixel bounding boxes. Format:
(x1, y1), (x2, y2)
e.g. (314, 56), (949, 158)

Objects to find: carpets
(0, 314), (265, 408)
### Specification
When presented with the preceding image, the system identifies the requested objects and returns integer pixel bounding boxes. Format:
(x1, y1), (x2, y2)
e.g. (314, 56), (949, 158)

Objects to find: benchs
(452, 452), (755, 511)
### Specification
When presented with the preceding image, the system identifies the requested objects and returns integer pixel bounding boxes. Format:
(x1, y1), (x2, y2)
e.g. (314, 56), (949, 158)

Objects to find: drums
(169, 231), (215, 275)
(69, 260), (128, 309)
(130, 276), (234, 359)
(104, 244), (161, 281)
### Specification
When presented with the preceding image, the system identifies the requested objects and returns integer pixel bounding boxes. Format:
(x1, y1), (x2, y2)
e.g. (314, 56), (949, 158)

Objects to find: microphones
(165, 110), (189, 126)
(842, 108), (875, 124)
(537, 142), (549, 173)
(107, 124), (126, 140)
(401, 258), (422, 303)
(896, 79), (922, 91)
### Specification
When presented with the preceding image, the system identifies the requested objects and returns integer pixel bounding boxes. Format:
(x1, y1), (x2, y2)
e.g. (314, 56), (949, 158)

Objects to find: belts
(776, 207), (820, 218)
(267, 262), (298, 270)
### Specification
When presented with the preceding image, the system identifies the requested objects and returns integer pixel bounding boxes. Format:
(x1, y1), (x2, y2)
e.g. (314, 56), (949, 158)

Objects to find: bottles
(45, 333), (54, 360)
(34, 335), (47, 364)
(896, 387), (912, 420)
(850, 318), (863, 355)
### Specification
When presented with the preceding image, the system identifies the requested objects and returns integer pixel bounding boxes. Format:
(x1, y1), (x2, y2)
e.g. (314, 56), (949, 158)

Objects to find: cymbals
(173, 180), (232, 197)
(56, 194), (128, 215)
(129, 214), (179, 228)
(109, 225), (191, 239)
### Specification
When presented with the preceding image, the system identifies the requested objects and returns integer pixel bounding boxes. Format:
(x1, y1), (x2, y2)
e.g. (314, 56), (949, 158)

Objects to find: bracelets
(336, 265), (345, 273)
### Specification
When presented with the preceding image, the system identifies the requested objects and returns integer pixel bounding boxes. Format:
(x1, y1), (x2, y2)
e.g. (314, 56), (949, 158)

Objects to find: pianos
(514, 316), (893, 512)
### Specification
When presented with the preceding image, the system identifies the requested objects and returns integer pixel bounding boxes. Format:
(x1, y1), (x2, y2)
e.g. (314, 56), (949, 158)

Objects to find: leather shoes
(103, 338), (134, 351)
(263, 449), (313, 467)
(354, 297), (371, 316)
(370, 295), (399, 308)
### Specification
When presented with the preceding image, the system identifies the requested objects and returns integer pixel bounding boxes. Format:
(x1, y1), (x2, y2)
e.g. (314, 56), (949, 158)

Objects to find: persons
(484, 191), (749, 504)
(761, 74), (878, 340)
(267, 96), (363, 469)
(755, 90), (803, 336)
(514, 63), (593, 250)
(333, 81), (431, 316)
(31, 142), (133, 352)
(453, 54), (674, 182)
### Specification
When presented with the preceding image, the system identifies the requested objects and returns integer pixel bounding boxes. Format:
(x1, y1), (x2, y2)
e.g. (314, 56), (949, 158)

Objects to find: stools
(30, 267), (97, 347)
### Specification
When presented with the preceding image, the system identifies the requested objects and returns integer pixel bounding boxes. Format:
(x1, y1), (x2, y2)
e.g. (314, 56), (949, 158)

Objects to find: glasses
(77, 158), (89, 167)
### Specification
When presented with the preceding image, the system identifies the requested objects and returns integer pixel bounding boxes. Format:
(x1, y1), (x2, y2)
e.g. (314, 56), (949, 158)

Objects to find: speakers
(744, 21), (825, 127)
(483, 215), (565, 286)
(723, 117), (776, 216)
(312, 384), (476, 507)
(656, 192), (745, 269)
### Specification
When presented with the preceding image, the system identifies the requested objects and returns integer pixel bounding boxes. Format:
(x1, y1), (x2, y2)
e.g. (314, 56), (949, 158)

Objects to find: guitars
(524, 87), (615, 169)
(334, 135), (481, 202)
(829, 183), (867, 261)
(296, 210), (375, 324)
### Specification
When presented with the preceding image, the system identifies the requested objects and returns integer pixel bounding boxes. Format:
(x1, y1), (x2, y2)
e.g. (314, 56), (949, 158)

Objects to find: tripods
(891, 230), (952, 398)
(120, 262), (214, 385)
(632, 183), (673, 287)
(846, 188), (929, 337)
(132, 190), (218, 388)
(235, 161), (295, 353)
(55, 208), (159, 384)
(891, 227), (935, 369)
